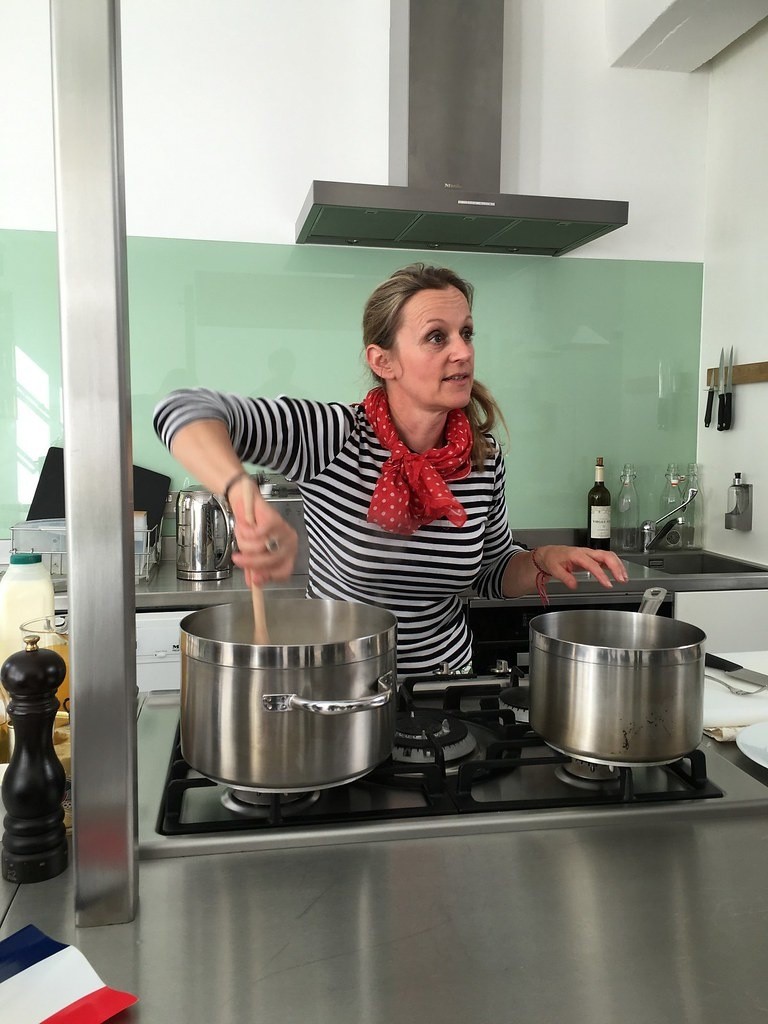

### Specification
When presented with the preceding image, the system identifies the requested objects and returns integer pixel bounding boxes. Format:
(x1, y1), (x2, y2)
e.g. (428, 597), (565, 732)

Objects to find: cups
(6, 719), (55, 763)
(20, 614), (70, 711)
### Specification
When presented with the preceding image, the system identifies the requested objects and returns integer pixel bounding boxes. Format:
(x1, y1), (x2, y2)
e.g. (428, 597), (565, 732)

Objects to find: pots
(527, 586), (707, 763)
(176, 598), (399, 785)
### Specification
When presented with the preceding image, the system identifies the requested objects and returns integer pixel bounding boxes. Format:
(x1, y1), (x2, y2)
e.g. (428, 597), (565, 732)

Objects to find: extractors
(296, 179), (628, 256)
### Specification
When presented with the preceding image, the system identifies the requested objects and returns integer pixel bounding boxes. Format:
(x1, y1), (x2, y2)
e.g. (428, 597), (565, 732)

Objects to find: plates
(735, 723), (768, 769)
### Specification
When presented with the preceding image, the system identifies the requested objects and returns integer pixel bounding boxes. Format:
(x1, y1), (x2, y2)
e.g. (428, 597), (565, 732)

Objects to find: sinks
(617, 553), (768, 575)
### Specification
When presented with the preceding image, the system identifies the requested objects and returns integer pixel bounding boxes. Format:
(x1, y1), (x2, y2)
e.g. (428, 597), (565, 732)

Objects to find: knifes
(717, 348), (725, 431)
(725, 345), (733, 430)
(704, 369), (714, 427)
(705, 652), (768, 686)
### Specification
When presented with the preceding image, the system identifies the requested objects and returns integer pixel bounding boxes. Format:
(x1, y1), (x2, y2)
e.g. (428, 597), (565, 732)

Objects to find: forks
(705, 675), (768, 695)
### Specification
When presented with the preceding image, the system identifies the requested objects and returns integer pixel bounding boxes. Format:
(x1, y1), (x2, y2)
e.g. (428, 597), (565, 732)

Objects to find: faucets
(638, 488), (699, 554)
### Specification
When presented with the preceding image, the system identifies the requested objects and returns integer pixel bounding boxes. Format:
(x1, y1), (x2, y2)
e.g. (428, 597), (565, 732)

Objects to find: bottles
(727, 473), (747, 529)
(587, 457), (611, 552)
(616, 463), (638, 552)
(0, 552), (54, 669)
(681, 462), (704, 550)
(656, 463), (681, 550)
(0, 635), (69, 884)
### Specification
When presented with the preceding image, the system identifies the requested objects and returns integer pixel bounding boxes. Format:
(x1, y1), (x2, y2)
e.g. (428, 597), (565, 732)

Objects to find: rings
(264, 536), (279, 553)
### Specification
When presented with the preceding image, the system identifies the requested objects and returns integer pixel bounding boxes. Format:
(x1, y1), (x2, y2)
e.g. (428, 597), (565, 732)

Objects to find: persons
(152, 261), (628, 702)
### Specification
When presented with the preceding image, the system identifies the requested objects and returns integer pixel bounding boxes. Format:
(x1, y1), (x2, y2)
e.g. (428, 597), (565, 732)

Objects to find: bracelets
(532, 549), (552, 606)
(225, 472), (260, 502)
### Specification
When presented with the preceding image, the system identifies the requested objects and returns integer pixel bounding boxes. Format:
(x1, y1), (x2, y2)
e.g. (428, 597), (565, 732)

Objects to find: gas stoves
(138, 659), (768, 861)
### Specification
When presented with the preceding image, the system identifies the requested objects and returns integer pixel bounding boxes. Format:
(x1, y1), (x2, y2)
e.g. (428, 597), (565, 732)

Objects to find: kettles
(175, 485), (235, 581)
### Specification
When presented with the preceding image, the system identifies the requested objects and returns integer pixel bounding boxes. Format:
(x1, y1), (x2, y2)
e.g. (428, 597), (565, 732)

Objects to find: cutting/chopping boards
(703, 650), (768, 728)
